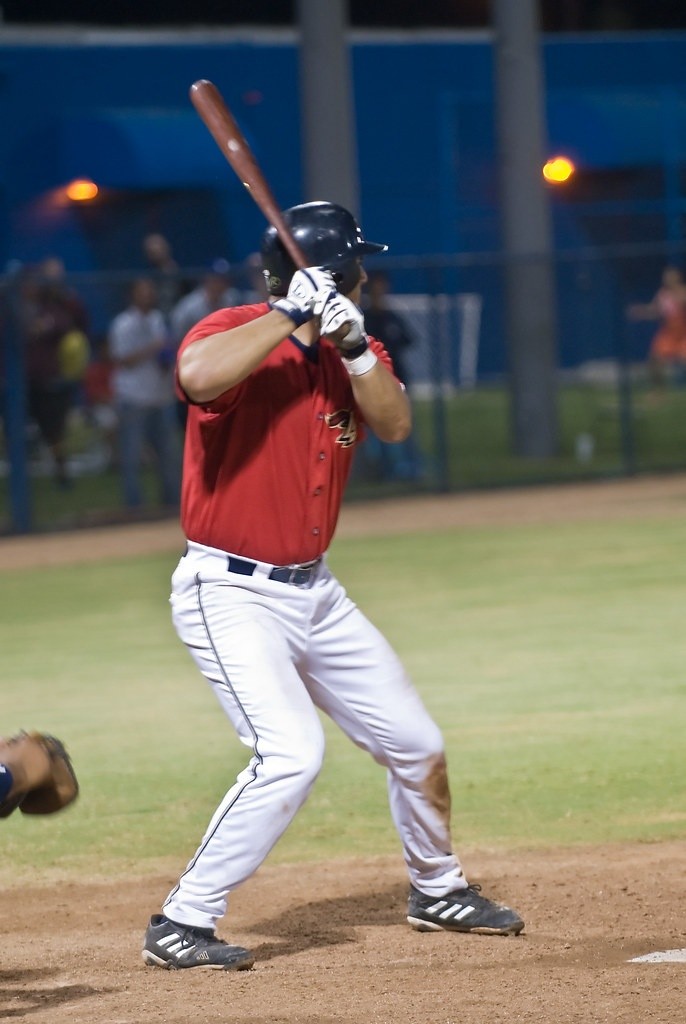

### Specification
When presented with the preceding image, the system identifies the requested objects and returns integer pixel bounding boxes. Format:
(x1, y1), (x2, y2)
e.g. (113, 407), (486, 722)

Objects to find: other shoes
(53, 455), (84, 486)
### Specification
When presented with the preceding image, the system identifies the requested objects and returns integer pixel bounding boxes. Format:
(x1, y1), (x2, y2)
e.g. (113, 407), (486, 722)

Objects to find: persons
(0, 726), (80, 820)
(11, 243), (419, 510)
(138, 196), (526, 971)
(624, 262), (686, 382)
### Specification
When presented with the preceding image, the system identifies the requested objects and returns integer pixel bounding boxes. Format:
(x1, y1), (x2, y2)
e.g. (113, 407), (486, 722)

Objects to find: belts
(228, 557), (322, 584)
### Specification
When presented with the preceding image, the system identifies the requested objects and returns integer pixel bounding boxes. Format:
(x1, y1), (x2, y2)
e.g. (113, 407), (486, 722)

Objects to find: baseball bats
(190, 79), (351, 338)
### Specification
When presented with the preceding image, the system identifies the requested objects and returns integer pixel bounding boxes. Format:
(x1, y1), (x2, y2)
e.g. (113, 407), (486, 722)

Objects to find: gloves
(271, 266), (337, 327)
(320, 292), (369, 359)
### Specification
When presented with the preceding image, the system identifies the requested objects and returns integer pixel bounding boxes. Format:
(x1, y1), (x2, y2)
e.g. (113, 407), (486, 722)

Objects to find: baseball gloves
(1, 728), (81, 815)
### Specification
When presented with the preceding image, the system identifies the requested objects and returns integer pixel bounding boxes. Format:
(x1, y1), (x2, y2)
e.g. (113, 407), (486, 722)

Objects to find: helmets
(261, 201), (386, 296)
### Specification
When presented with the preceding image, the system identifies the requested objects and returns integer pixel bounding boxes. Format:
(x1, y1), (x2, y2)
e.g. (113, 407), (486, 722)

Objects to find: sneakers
(142, 913), (257, 971)
(407, 882), (525, 933)
